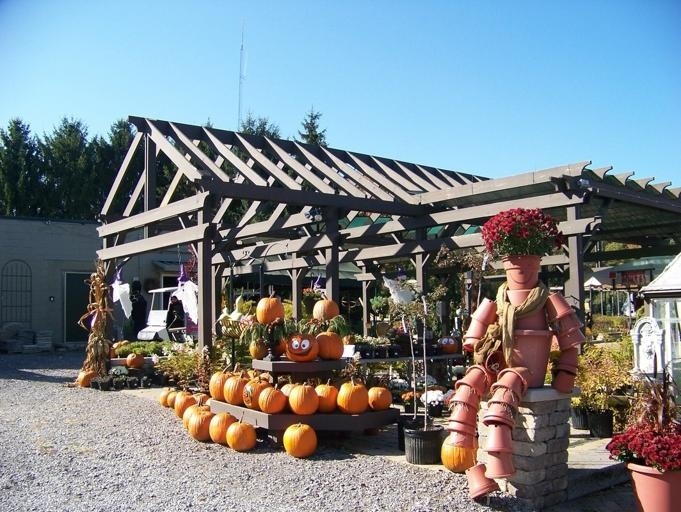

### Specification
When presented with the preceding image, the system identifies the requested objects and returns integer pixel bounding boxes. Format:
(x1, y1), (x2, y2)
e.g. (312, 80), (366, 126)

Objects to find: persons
(131, 290), (148, 331)
(167, 295), (183, 327)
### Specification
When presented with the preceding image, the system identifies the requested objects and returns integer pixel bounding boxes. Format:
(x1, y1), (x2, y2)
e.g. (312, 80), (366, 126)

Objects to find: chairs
(20, 329), (54, 354)
(7, 330), (34, 353)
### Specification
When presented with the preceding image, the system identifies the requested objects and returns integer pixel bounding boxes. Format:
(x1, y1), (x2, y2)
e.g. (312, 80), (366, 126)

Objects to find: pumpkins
(313, 299), (340, 322)
(440, 434), (477, 472)
(126, 353), (144, 368)
(159, 332), (393, 458)
(255, 297), (285, 324)
(78, 369), (99, 387)
(438, 336), (458, 354)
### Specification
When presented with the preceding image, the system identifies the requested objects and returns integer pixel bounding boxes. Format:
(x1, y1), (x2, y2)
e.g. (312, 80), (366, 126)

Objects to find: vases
(627, 460), (681, 512)
(503, 255), (542, 290)
(448, 287), (587, 499)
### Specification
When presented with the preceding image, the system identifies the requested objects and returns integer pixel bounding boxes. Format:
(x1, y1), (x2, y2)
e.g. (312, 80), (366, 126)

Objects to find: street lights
(464, 271), (475, 317)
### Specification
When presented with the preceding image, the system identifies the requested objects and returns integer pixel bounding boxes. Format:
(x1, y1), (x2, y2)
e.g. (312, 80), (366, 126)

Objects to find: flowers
(356, 334), (469, 418)
(151, 333), (215, 393)
(607, 416), (681, 472)
(478, 206), (562, 258)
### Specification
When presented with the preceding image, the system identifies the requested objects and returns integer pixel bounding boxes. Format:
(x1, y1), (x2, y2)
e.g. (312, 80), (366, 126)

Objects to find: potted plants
(393, 278), (449, 466)
(371, 296), (391, 336)
(546, 336), (636, 437)
(389, 301), (439, 451)
(91, 375), (152, 390)
